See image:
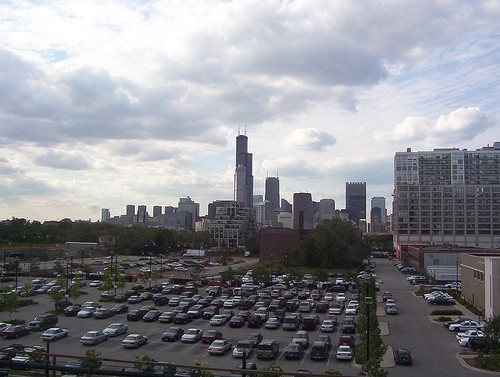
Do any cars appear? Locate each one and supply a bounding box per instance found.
[207,339,232,356]
[10,346,44,364]
[122,333,148,350]
[40,327,69,342]
[357,261,377,279]
[336,335,356,361]
[235,362,313,377]
[102,323,129,337]
[443,317,487,345]
[230,332,263,358]
[383,291,398,316]
[0,322,12,331]
[308,334,333,362]
[423,290,457,306]
[394,348,413,366]
[11,255,361,344]
[78,329,109,346]
[444,281,462,288]
[376,280,384,291]
[0,325,31,340]
[283,330,310,360]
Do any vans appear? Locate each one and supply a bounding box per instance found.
[396,263,416,275]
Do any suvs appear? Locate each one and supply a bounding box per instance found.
[257,338,280,361]
[405,275,425,281]
[28,313,59,332]
